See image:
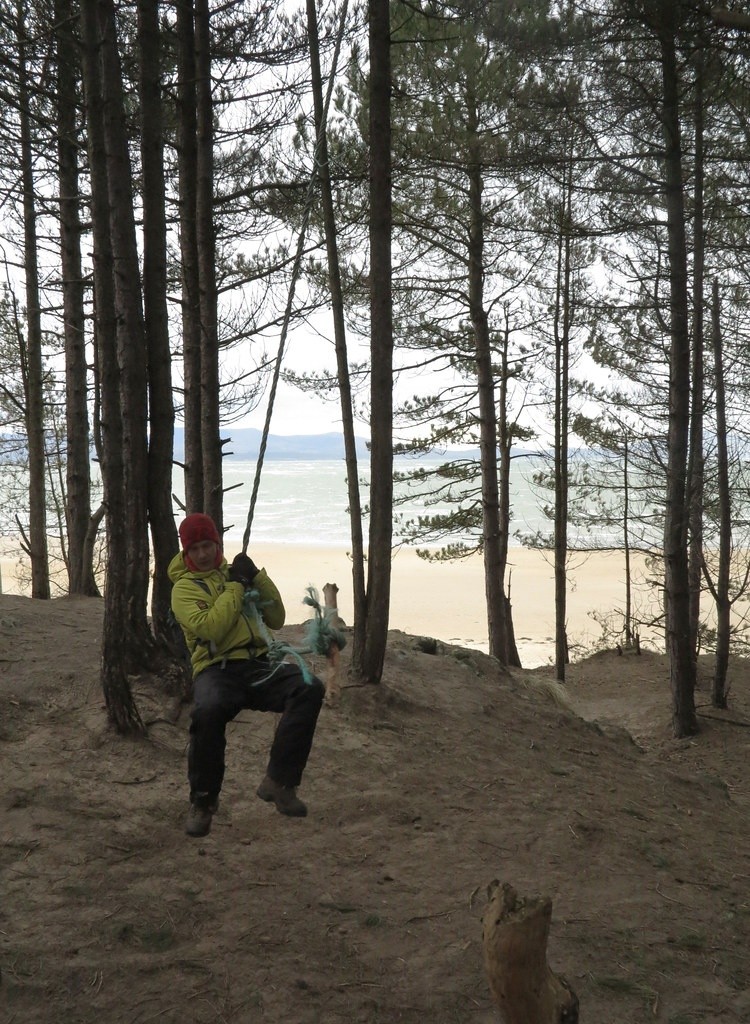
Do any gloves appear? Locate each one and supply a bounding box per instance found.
[233,553,260,579]
[228,565,249,590]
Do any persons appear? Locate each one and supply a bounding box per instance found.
[167,509,330,839]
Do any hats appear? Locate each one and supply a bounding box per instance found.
[178,513,220,552]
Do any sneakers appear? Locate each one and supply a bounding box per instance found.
[185,792,221,839]
[255,775,308,818]
